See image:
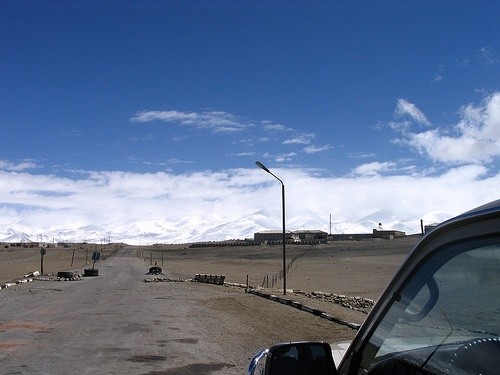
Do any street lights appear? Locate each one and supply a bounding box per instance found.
[255,161,287,295]
